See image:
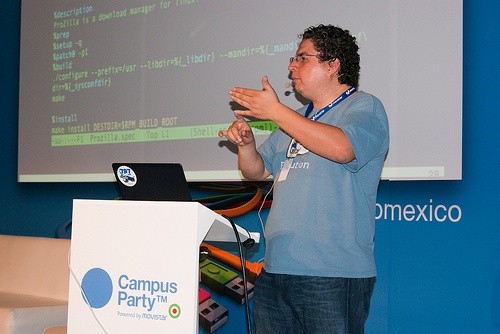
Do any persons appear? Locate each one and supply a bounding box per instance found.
[218,24,390,334]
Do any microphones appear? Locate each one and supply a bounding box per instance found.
[284,82,295,88]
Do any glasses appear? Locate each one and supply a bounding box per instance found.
[290,54,321,64]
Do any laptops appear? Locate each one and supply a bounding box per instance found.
[112,163,192,202]
